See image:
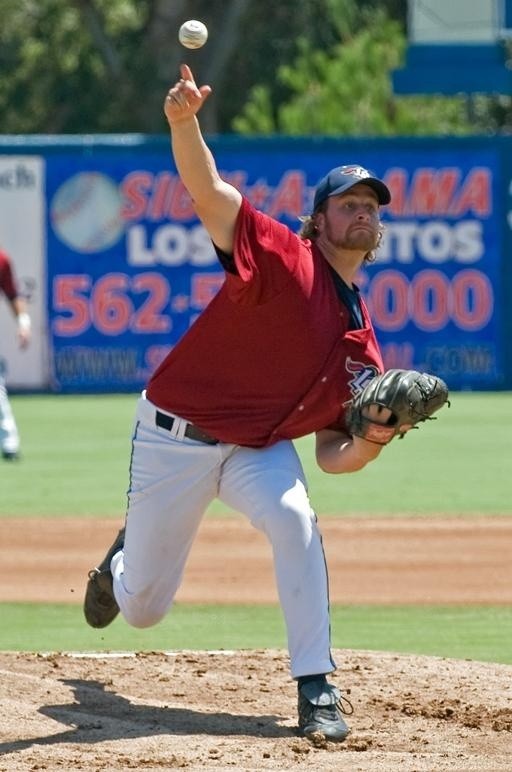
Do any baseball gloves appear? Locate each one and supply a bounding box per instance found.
[346,365,448,445]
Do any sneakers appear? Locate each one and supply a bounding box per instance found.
[84,528,125,629]
[297,681,348,741]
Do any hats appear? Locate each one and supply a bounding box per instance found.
[313,164,392,207]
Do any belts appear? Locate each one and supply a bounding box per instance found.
[155,411,217,445]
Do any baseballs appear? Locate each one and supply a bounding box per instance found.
[178,17,210,50]
[49,171,129,254]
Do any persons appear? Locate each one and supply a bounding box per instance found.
[86,64,450,741]
[2,248,32,459]
